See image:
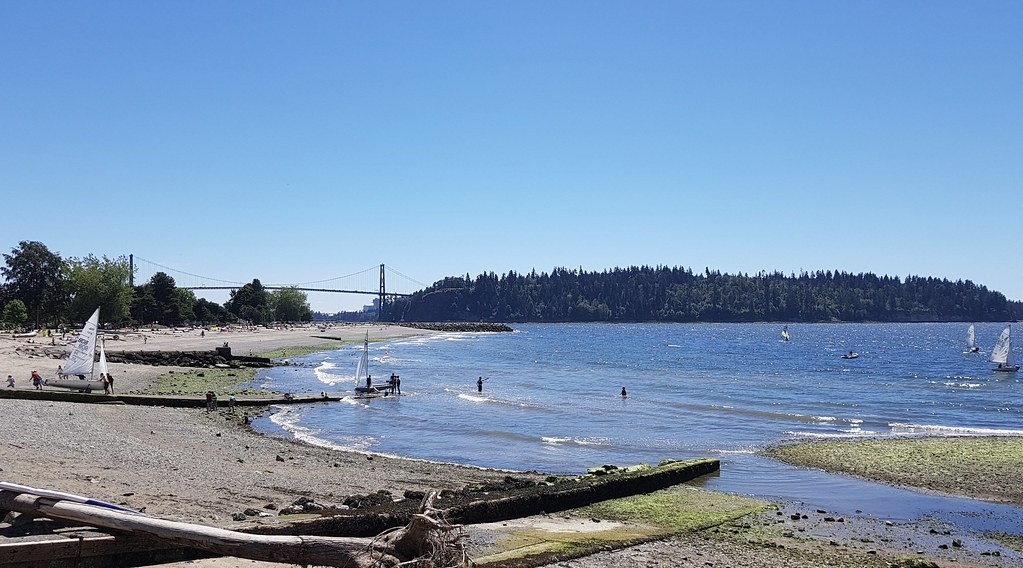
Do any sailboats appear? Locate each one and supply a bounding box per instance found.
[961,323,979,354]
[42,306,112,390]
[353,328,392,391]
[987,323,1022,371]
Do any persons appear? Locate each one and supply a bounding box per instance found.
[284,359,291,366]
[848,350,853,357]
[367,375,372,393]
[389,373,401,395]
[75,374,87,380]
[228,393,236,412]
[267,320,395,331]
[998,363,1008,368]
[55,365,68,380]
[96,373,110,395]
[206,391,218,414]
[786,337,788,341]
[619,387,627,396]
[29,370,45,390]
[6,374,15,388]
[107,373,114,394]
[476,376,483,392]
[5,320,262,345]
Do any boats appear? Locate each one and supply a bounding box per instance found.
[841,352,859,359]
